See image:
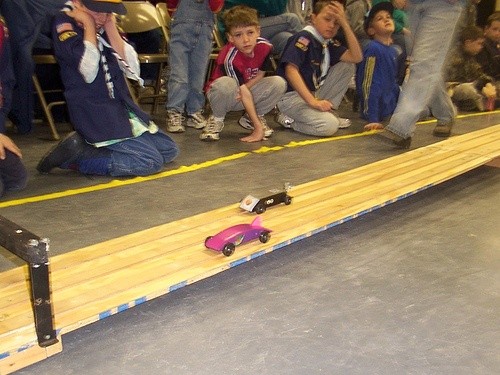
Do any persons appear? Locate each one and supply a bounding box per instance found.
[198,0,500,149]
[1,0,179,199]
[165,0,224,132]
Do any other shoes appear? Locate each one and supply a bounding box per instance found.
[7,107,32,134]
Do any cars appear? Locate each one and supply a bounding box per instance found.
[239,186,293,215]
[204,217,272,257]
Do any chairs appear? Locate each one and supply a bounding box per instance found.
[30,1,222,141]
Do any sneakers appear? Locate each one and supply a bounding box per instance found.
[184,111,207,129]
[273,112,293,129]
[198,114,224,142]
[36,130,93,173]
[239,114,273,137]
[374,129,411,149]
[165,109,186,132]
[433,113,453,137]
[338,116,351,128]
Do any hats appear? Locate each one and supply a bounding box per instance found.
[363,1,393,30]
[81,0,128,15]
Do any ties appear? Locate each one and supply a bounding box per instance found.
[300,24,333,78]
